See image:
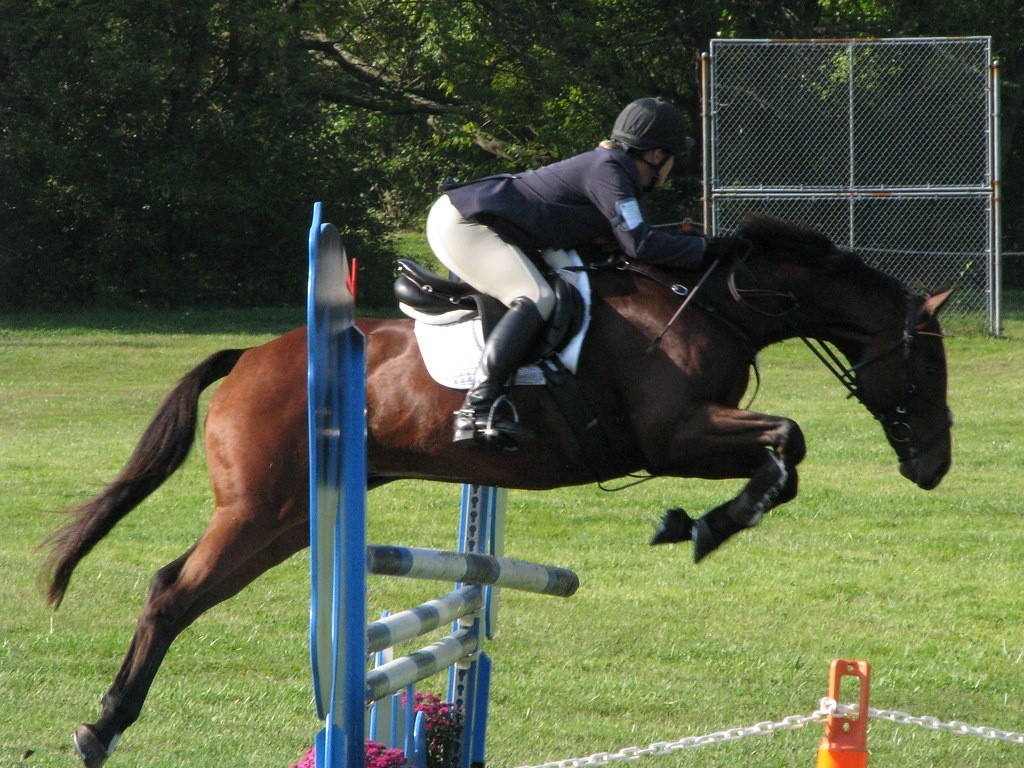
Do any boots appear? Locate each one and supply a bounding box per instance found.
[451,296,536,446]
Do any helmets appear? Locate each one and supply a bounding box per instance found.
[611,95,695,156]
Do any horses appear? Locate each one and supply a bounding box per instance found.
[30,214,955,768]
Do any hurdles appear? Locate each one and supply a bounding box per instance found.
[307,199,578,766]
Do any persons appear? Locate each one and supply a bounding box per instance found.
[427,99,749,445]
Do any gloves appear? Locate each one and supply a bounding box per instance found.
[704,234,750,264]
